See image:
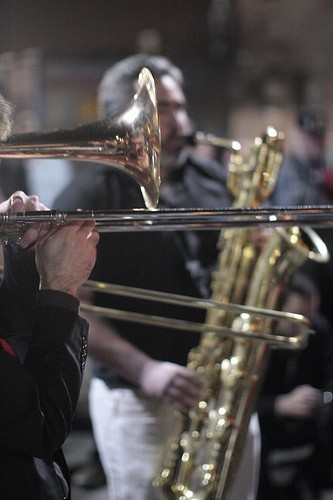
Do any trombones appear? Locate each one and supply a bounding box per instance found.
[73,279,312,350]
[0,67,333,234]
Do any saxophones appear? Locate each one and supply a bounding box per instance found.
[157,125,330,499]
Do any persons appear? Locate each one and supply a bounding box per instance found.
[1,95,102,500]
[197,96,333,500]
[51,51,286,500]
